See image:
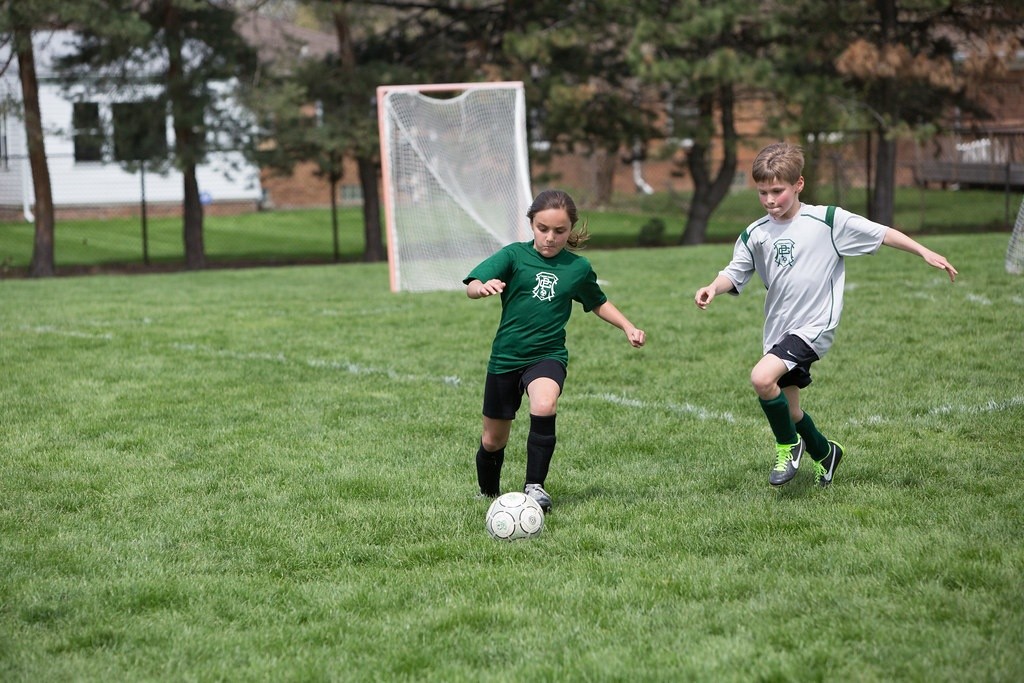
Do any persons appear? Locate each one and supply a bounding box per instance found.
[694,139,959,491]
[463,190,648,508]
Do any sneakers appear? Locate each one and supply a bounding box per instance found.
[813,440,845,487]
[524,483,552,513]
[768,433,806,487]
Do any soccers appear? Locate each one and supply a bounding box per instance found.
[485,492,547,542]
